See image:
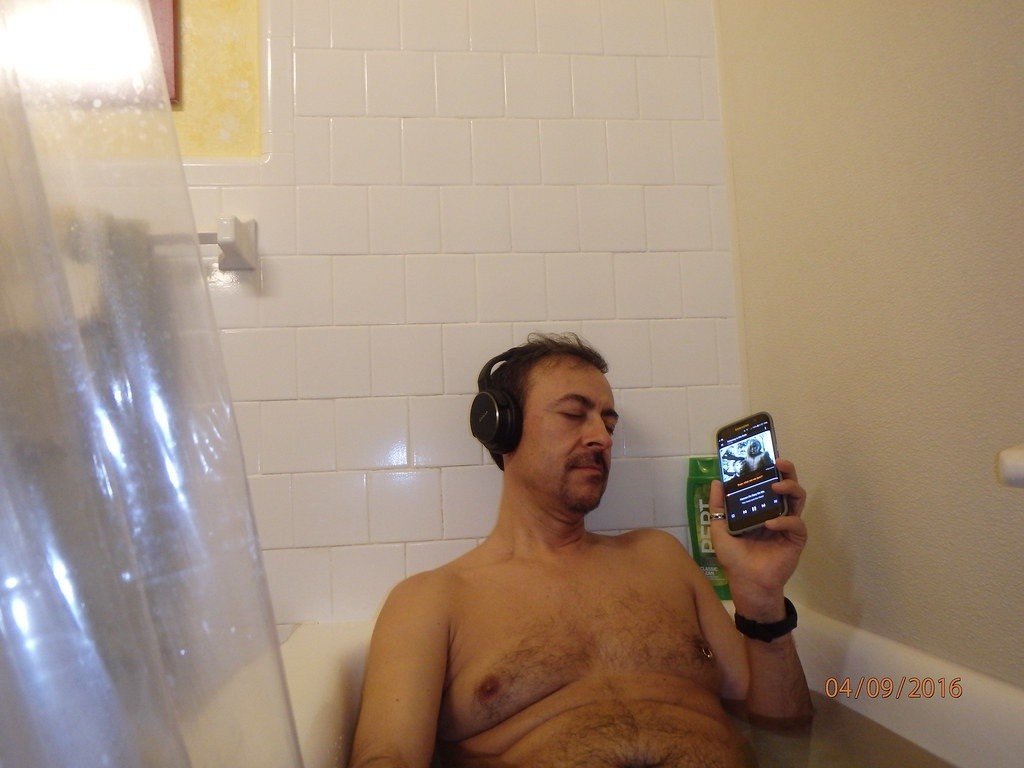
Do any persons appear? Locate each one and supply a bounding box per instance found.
[744,439,771,473]
[351,337,812,768]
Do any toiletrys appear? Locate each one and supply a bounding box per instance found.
[685,455,732,603]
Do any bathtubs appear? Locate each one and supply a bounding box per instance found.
[189,591,1024,768]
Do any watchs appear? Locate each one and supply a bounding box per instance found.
[734,597,797,642]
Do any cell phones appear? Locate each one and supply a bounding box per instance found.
[718,411,790,536]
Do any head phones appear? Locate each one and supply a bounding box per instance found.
[469,346,527,455]
